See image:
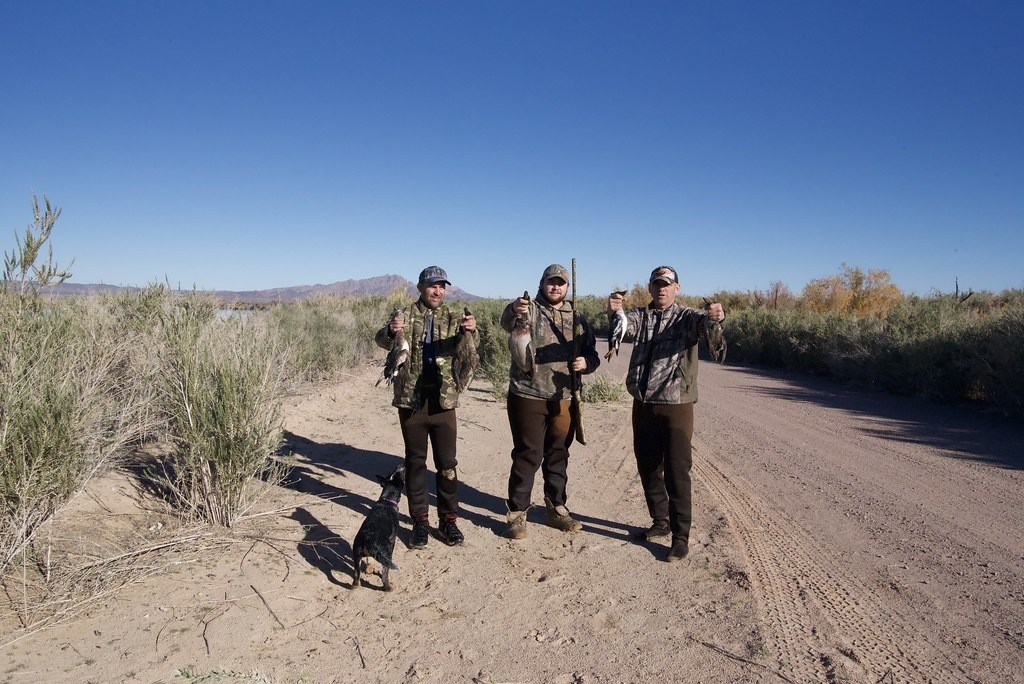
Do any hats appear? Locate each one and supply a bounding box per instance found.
[544,264,568,283]
[651,266,678,284]
[419,265,451,286]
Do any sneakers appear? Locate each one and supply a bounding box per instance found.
[409,512,430,548]
[438,516,465,546]
[629,526,670,544]
[505,500,534,539]
[543,497,582,530]
[667,541,688,562]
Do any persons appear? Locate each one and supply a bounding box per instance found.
[499,263,600,540]
[604,265,726,562]
[373,265,481,549]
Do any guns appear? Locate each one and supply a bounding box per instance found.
[570,258,587,446]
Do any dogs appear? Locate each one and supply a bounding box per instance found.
[350,463,406,593]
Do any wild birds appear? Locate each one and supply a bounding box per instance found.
[507,290,537,381]
[702,297,728,365]
[374,307,410,390]
[604,290,628,363]
[451,307,480,394]
